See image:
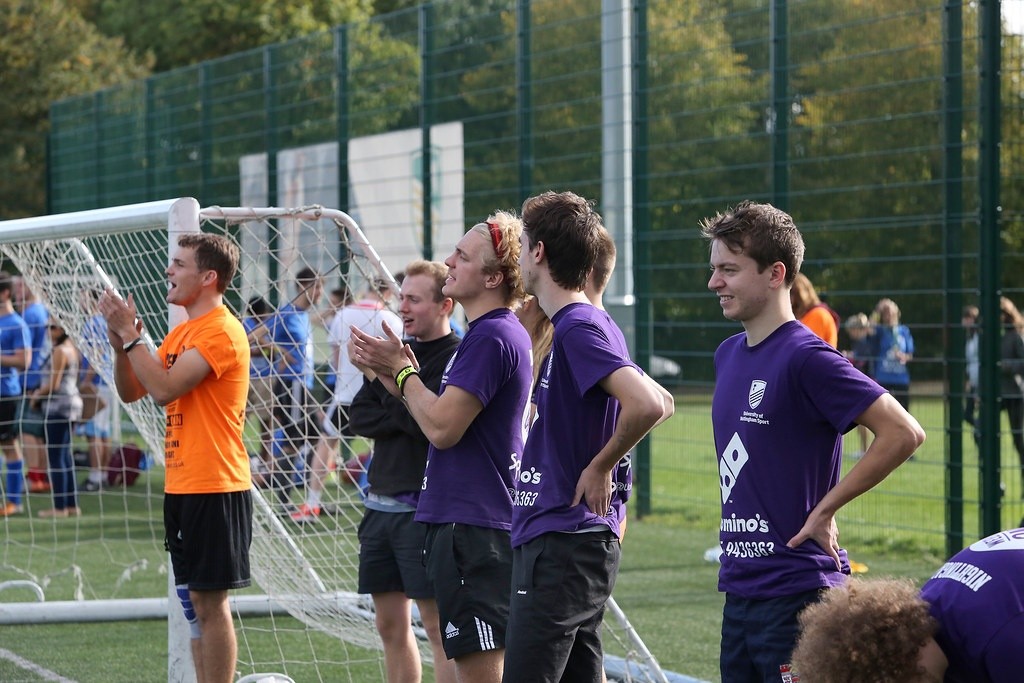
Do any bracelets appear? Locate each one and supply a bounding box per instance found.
[395,365,422,396]
[123,337,145,353]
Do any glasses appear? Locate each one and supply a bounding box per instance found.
[45,323,61,331]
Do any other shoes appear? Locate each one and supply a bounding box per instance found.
[39,503,82,517]
[21,478,50,492]
[78,477,109,491]
[0,502,24,517]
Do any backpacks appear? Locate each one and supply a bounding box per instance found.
[108,442,144,488]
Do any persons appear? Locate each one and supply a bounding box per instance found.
[789,273,915,459]
[501,191,673,683]
[349,209,532,683]
[960,297,1024,527]
[96,233,253,683]
[699,199,926,683]
[0,268,118,518]
[243,267,465,524]
[349,260,463,683]
[790,528,1023,683]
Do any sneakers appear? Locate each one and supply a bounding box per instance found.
[292,504,319,523]
